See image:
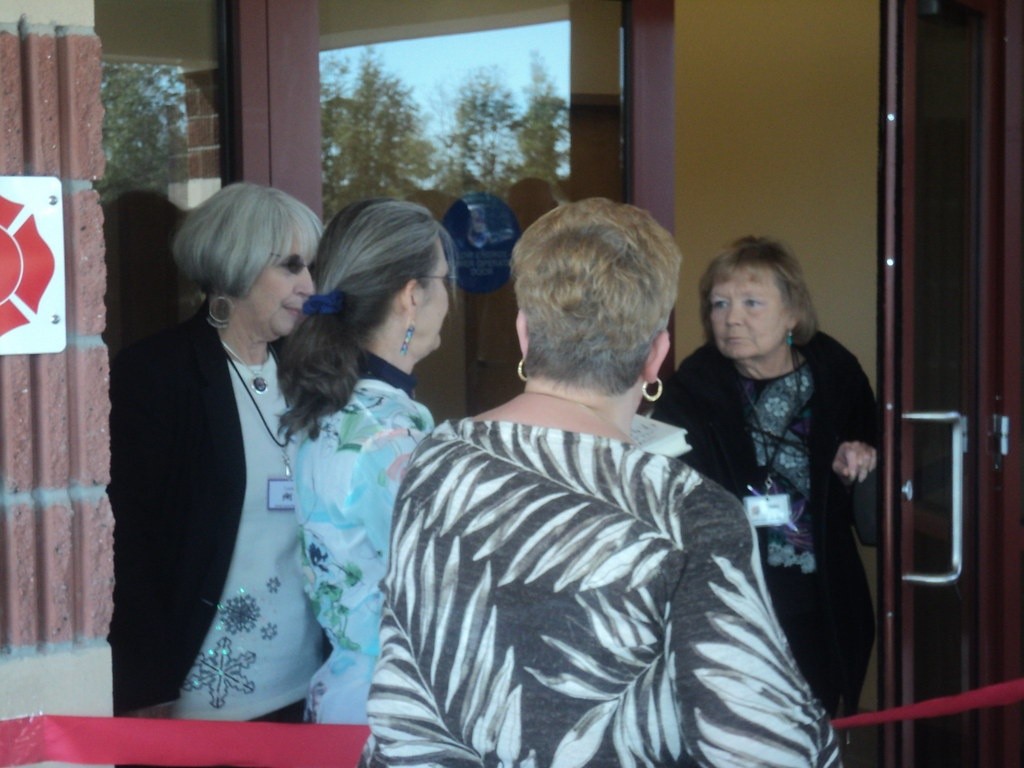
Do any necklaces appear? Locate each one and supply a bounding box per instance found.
[221,337,269,393]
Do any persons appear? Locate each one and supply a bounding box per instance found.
[304,196,848,768]
[109,181,332,768]
[275,197,455,725]
[651,234,883,719]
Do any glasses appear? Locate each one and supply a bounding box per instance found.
[271,250,319,280]
[417,266,460,307]
